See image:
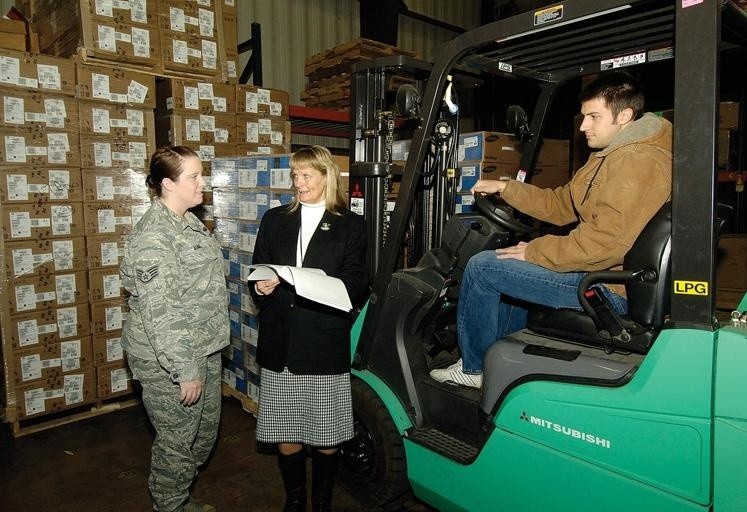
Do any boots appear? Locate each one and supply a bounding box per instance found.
[276,446,307,512]
[310,448,342,512]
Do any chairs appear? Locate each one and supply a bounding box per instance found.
[479,201,734,417]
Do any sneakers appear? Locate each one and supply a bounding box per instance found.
[183,496,217,512]
[427,356,483,388]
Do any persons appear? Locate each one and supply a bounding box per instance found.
[117,143,233,511]
[428,68,673,396]
[246,143,373,510]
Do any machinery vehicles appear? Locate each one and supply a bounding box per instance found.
[335,0,746,512]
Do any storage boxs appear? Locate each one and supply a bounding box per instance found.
[664,99,746,310]
[1,0,569,422]
